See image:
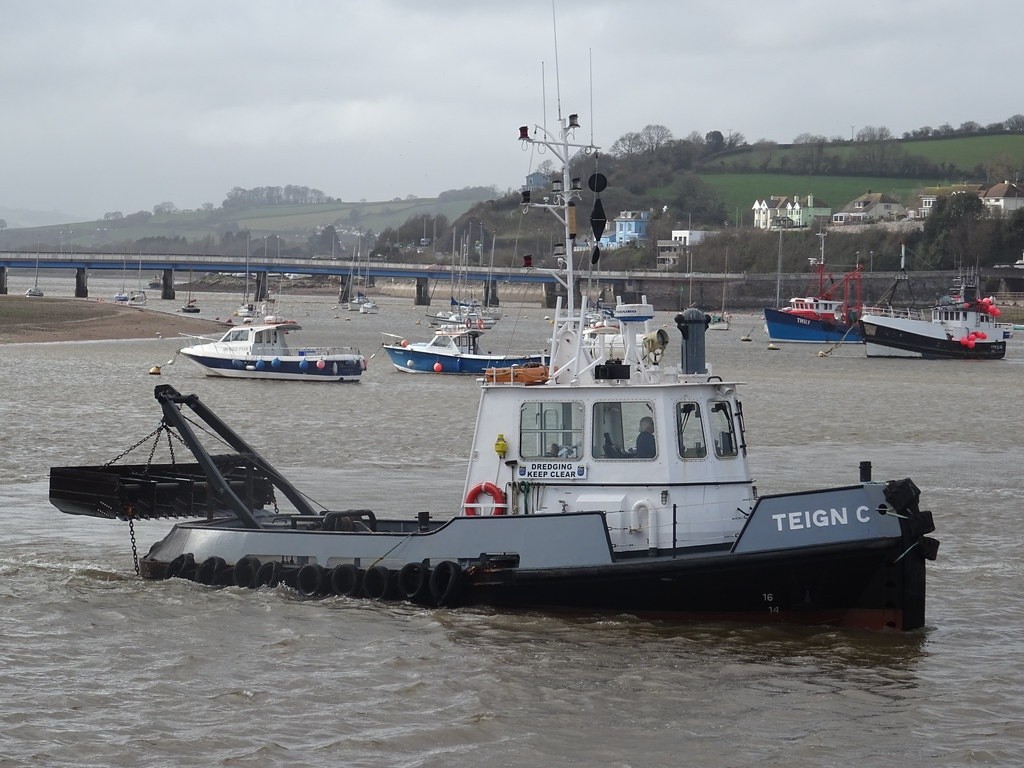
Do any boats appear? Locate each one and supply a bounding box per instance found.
[762,259,867,344]
[48,0,940,630]
[382,300,550,376]
[180,316,367,382]
[857,255,1014,359]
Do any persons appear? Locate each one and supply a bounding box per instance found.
[636,417,656,458]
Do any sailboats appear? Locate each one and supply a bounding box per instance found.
[19,214,751,330]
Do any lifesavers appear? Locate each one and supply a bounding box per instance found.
[658,446,778,547]
[161,552,463,609]
[476,318,483,329]
[465,317,472,329]
[463,481,505,515]
